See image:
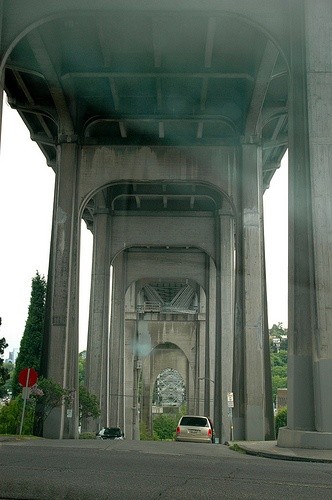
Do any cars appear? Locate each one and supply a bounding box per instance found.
[95,427,124,440]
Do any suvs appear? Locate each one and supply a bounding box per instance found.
[175,415,214,443]
